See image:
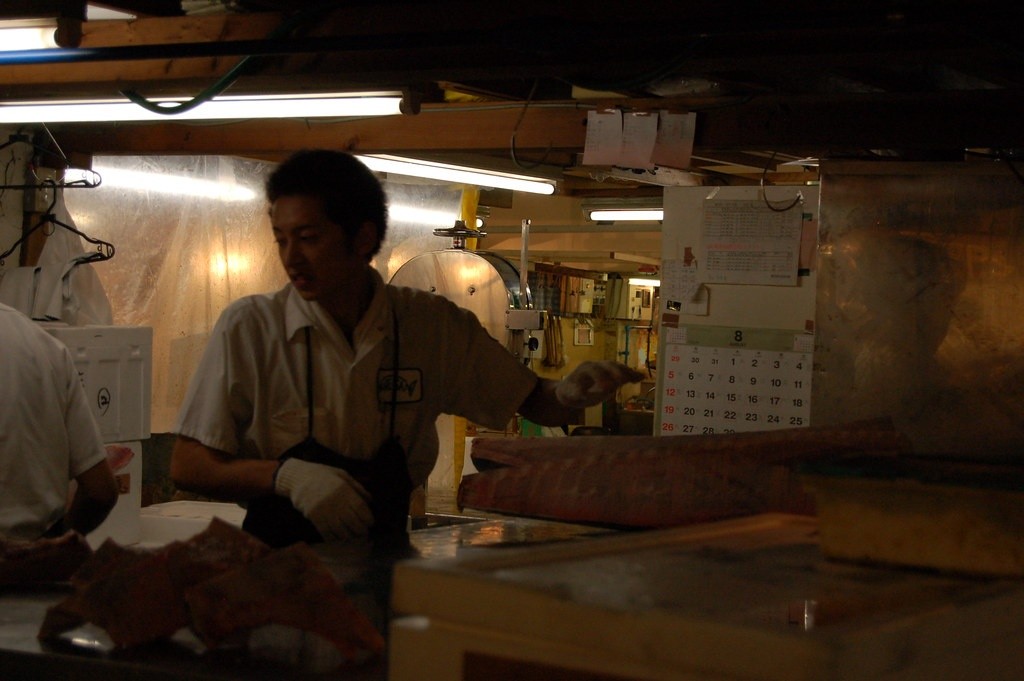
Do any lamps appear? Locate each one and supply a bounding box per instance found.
[354,152,565,198]
[579,203,664,223]
[0,74,422,128]
[0,14,83,51]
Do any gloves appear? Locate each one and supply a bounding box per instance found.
[555,360,645,409]
[275,457,375,543]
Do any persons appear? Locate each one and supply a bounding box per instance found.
[1,300,119,540]
[169,147,647,549]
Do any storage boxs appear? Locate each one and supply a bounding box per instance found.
[42,322,152,444]
[69,442,142,512]
